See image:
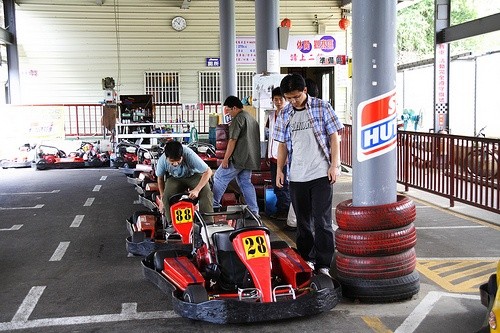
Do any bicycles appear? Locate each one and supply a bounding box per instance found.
[464,126,500,180]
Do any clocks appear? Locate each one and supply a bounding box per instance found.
[172,16,187,31]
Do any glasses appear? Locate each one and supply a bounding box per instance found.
[286,90,303,102]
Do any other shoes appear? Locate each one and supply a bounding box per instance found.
[270,212,280,218]
[281,223,297,232]
[245,216,260,220]
[307,261,314,271]
[277,215,287,220]
[318,268,331,277]
[214,208,220,212]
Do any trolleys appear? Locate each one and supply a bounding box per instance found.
[411,128,450,170]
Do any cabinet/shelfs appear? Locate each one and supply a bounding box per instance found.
[120,95,153,145]
[116,123,195,149]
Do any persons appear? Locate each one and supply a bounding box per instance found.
[155,140,213,225]
[264,87,292,222]
[212,95,261,225]
[273,73,344,279]
